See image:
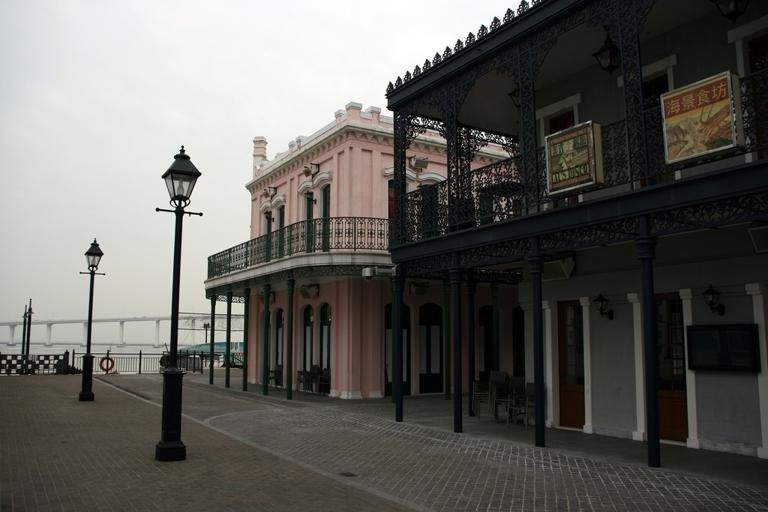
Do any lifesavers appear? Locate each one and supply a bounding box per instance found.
[100,357,114,371]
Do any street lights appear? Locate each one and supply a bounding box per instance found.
[79,238,106,401]
[156,145,204,460]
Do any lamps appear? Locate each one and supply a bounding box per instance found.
[508,87,521,110]
[593,35,621,77]
[593,290,614,320]
[264,209,276,222]
[303,189,316,205]
[701,283,726,316]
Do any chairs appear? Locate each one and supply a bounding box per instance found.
[265,365,281,391]
[470,369,547,432]
[294,370,330,398]
